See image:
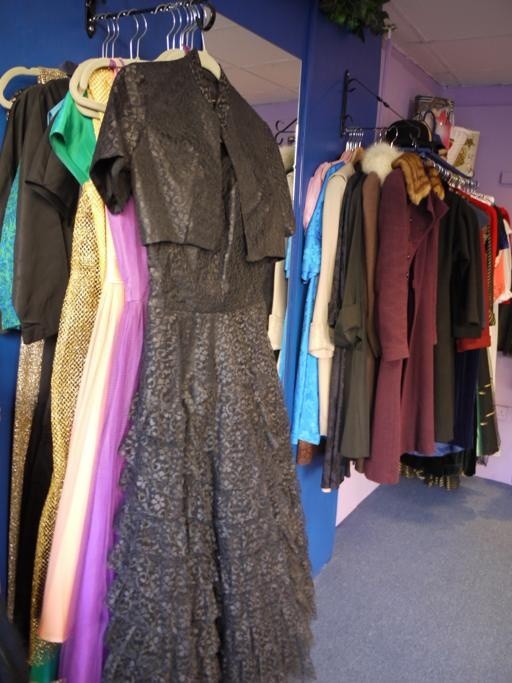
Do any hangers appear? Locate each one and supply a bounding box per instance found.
[345,126,479,194]
[89,0,209,58]
